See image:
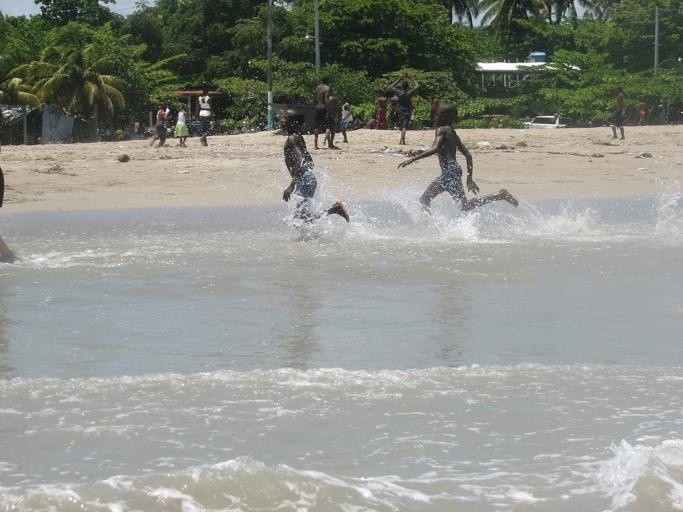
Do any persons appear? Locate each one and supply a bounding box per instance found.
[192,87,215,148]
[608,86,628,142]
[149,105,172,149]
[0,165,14,263]
[395,99,519,220]
[173,104,195,148]
[636,98,648,127]
[276,107,350,245]
[305,72,419,150]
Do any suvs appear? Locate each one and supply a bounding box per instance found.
[523,115,568,128]
